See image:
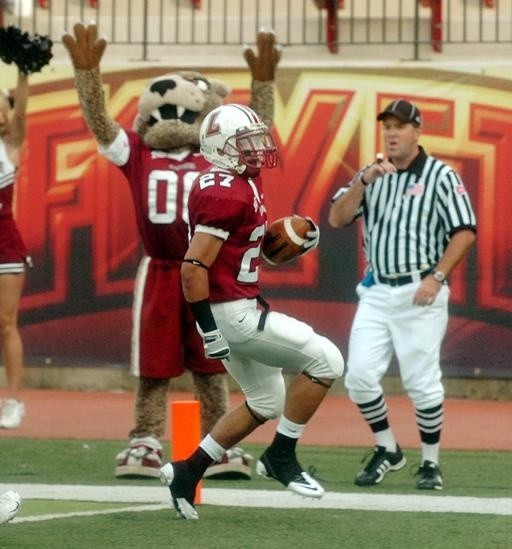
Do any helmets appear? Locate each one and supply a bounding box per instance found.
[198,103,279,177]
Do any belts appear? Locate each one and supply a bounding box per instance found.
[375,270,435,289]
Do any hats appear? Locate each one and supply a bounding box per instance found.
[376,99,422,126]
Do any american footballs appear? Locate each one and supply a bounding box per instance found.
[260,217,311,267]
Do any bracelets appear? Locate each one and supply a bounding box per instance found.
[361,176,367,186]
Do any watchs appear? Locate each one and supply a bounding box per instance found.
[428,268,447,286]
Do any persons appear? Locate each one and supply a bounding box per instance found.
[1,488,24,526]
[155,99,347,522]
[1,67,36,431]
[324,99,482,496]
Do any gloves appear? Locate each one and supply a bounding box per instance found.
[293,212,321,258]
[200,328,232,362]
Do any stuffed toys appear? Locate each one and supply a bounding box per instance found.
[60,19,284,481]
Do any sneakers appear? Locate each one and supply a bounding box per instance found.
[159,461,201,520]
[0,489,21,524]
[255,446,325,499]
[354,443,407,487]
[203,447,252,481]
[414,459,443,490]
[0,397,25,429]
[113,436,164,478]
